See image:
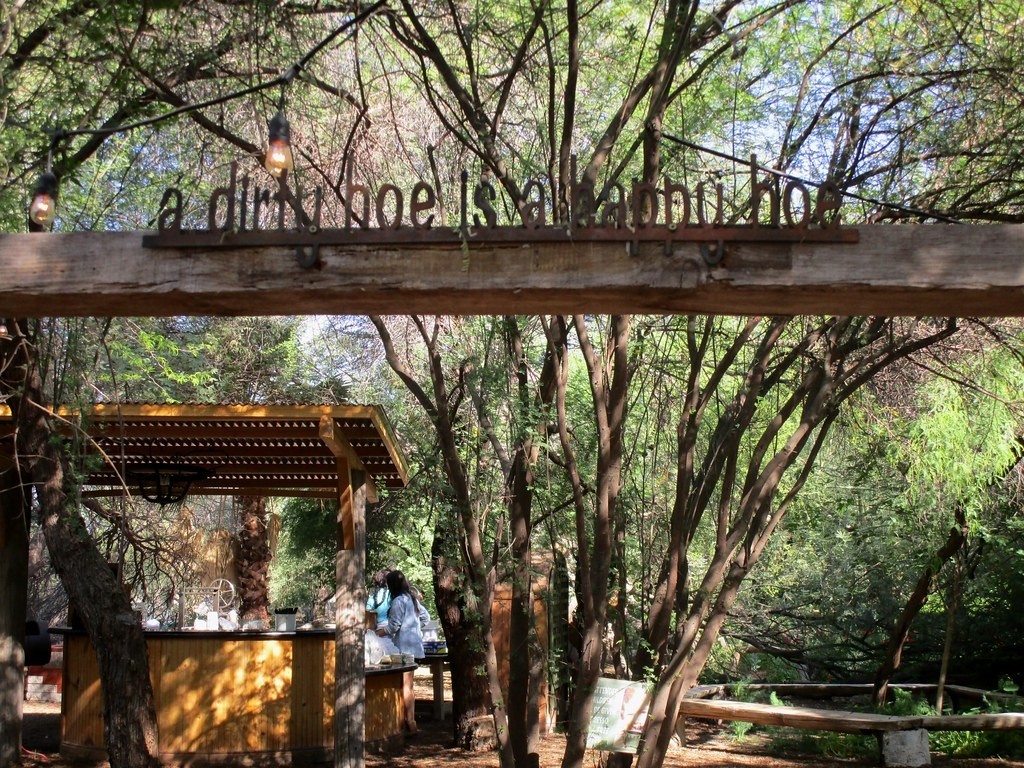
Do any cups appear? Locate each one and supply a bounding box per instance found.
[207,611,218,630]
[275,614,296,631]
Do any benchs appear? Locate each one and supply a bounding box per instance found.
[675,683,1024,768]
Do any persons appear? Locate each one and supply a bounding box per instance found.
[365,570,430,736]
[227,607,239,631]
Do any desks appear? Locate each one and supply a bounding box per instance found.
[365,662,418,675]
[424,654,453,720]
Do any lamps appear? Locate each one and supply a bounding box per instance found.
[125,463,211,507]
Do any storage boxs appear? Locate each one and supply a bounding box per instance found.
[423,641,448,654]
